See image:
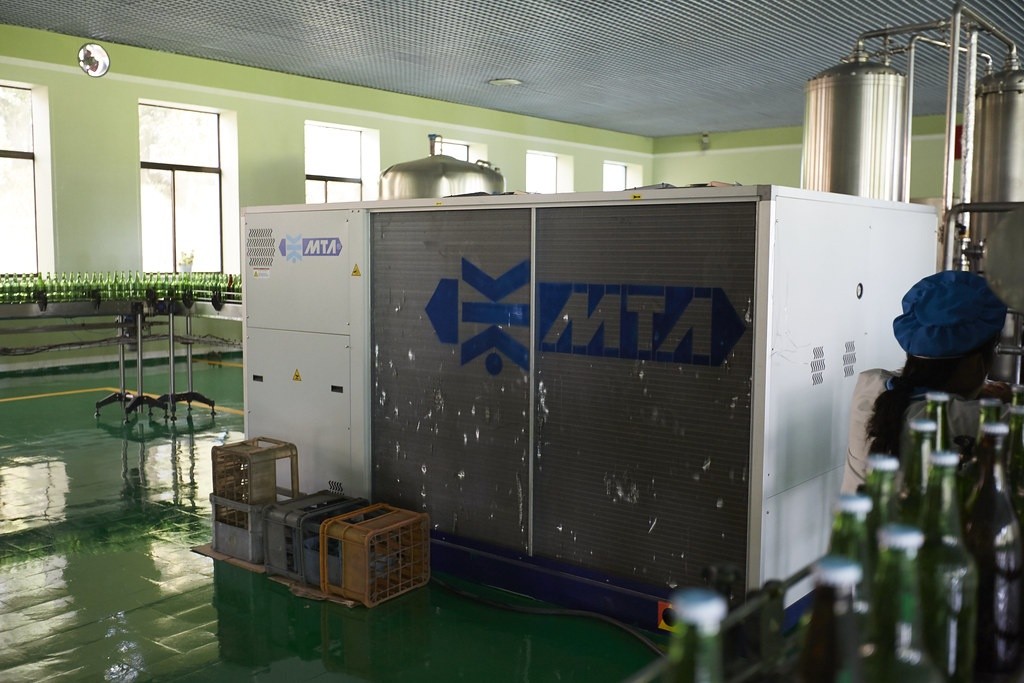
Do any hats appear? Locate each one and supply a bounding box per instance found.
[892,271,1007,358]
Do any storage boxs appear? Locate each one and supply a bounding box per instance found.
[211,437,431,609]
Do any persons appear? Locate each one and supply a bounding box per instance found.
[838,268,1013,496]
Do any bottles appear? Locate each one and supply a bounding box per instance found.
[865,419,935,524]
[193,272,242,300]
[974,398,1002,457]
[36,272,45,302]
[28,273,36,303]
[53,272,61,302]
[98,272,105,301]
[119,271,141,299]
[142,272,192,299]
[45,272,53,302]
[803,560,863,683]
[76,272,83,302]
[61,272,68,302]
[669,589,727,683]
[20,273,28,302]
[68,272,76,302]
[0,273,20,303]
[965,423,1024,683]
[925,393,951,454]
[920,451,977,683]
[90,272,98,301]
[105,271,120,300]
[1006,385,1024,543]
[83,272,90,301]
[826,496,943,683]
